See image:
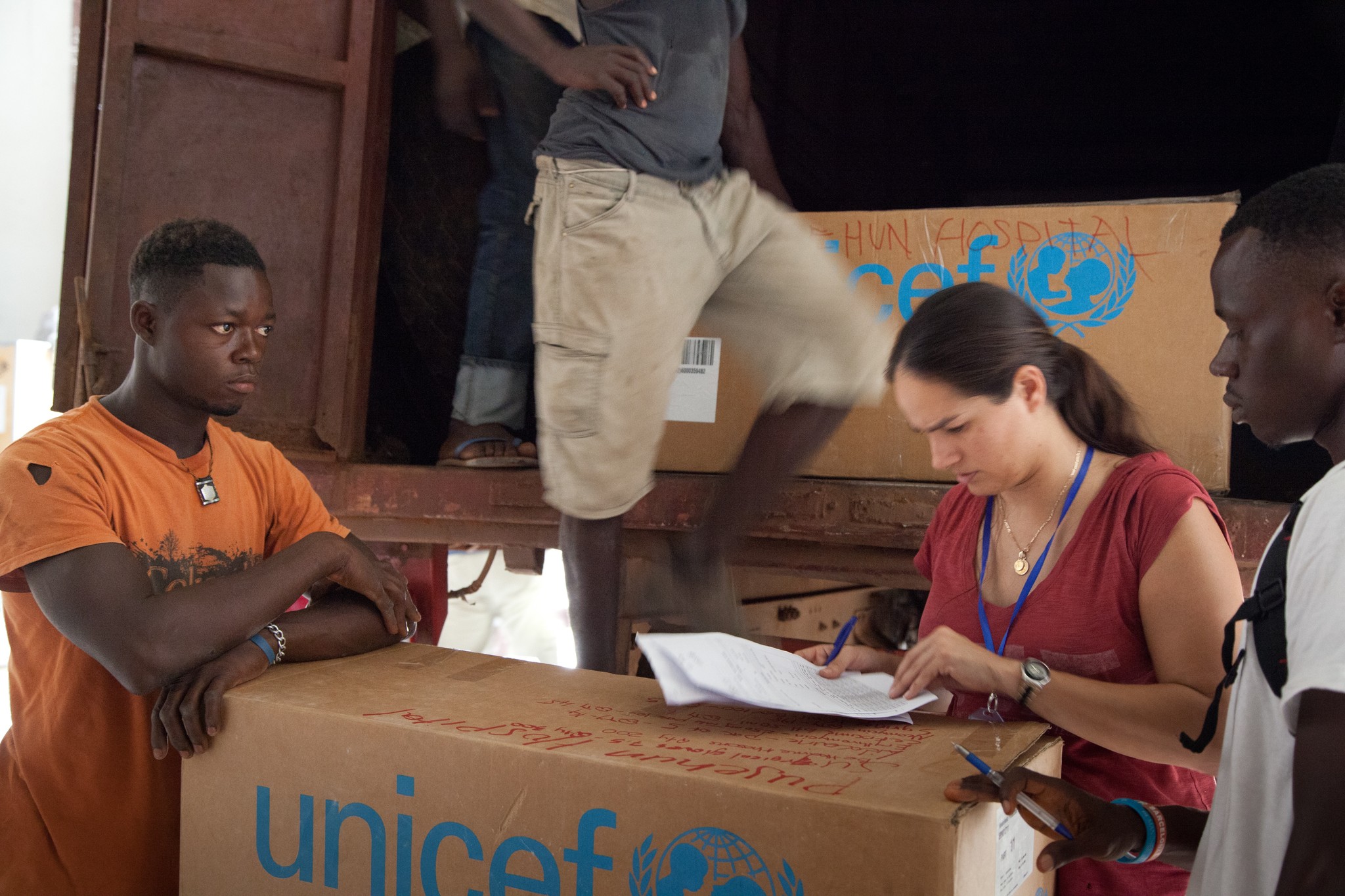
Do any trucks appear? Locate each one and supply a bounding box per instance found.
[67,1,1292,687]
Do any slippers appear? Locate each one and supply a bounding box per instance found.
[432,435,539,471]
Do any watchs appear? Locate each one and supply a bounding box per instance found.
[1016,656,1051,707]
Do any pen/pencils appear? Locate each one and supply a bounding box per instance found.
[950,740,1073,840]
[822,609,861,667]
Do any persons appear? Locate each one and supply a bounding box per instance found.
[793,282,1246,896]
[941,161,1345,896]
[0,217,424,896]
[424,0,580,467]
[458,0,888,675]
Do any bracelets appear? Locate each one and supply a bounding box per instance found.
[1127,800,1166,863]
[1109,798,1156,864]
[251,634,276,667]
[266,620,287,666]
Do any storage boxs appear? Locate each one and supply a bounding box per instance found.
[184,636,1064,896]
[648,196,1238,498]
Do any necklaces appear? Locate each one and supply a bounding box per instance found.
[169,428,220,506]
[997,437,1080,575]
